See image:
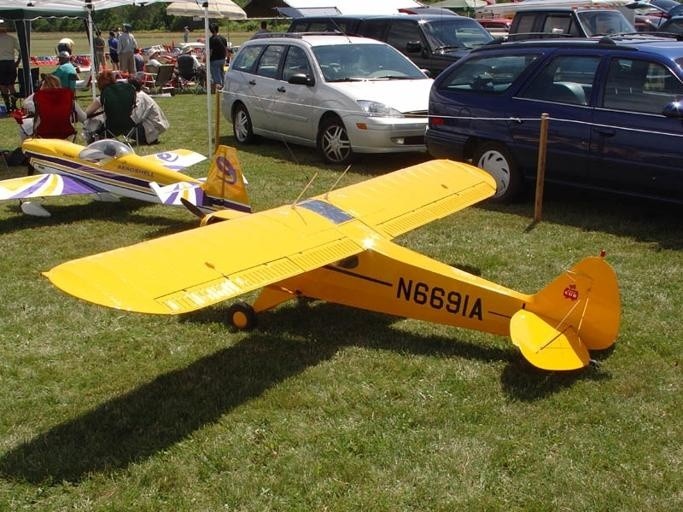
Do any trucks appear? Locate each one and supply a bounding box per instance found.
[481,0,627,18]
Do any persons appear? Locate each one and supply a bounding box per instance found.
[0,23,272,141]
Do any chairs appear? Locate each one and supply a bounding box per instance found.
[335,48,369,79]
[178,56,207,95]
[605,65,657,115]
[32,86,78,144]
[141,64,175,95]
[11,66,39,116]
[100,83,140,146]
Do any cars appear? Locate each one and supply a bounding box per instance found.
[423,30,683,204]
[221,29,439,164]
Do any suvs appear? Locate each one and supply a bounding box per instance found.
[507,7,638,41]
[474,17,512,40]
[627,0,682,38]
[287,15,495,78]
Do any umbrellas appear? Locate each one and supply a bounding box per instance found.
[165,0,247,20]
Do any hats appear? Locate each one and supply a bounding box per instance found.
[55,50,69,59]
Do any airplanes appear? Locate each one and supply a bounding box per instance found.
[45,158,621,389]
[0,136,253,227]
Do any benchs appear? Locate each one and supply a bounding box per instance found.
[523,63,588,106]
